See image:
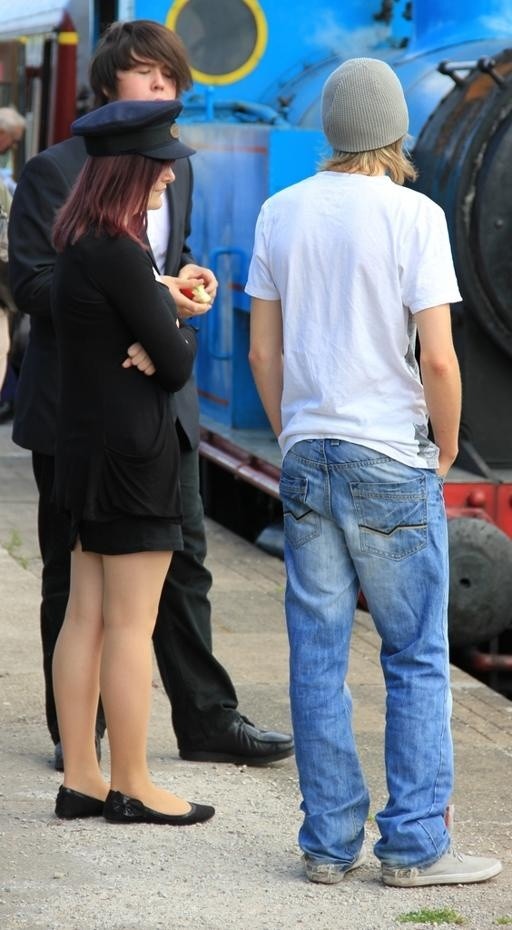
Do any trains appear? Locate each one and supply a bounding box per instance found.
[2,2,512,671]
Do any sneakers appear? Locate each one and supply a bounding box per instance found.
[382,847,504,887]
[304,846,367,884]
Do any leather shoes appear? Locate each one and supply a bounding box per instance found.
[55,734,101,771]
[179,717,295,766]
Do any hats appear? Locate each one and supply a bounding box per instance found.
[321,58,409,153]
[71,100,197,161]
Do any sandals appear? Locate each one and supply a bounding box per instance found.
[55,784,215,825]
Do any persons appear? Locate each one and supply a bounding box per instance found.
[241,55,503,888]
[8,18,298,767]
[0,102,28,428]
[48,95,218,827]
